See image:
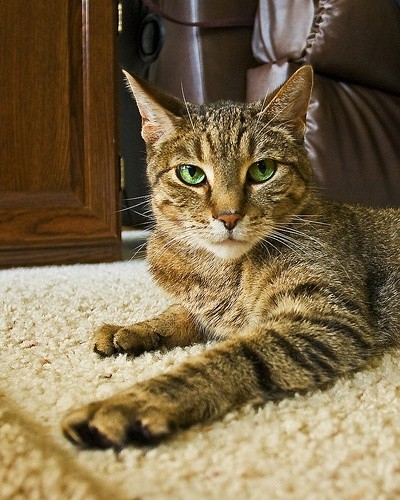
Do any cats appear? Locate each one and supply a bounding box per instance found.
[60,63,400,453]
